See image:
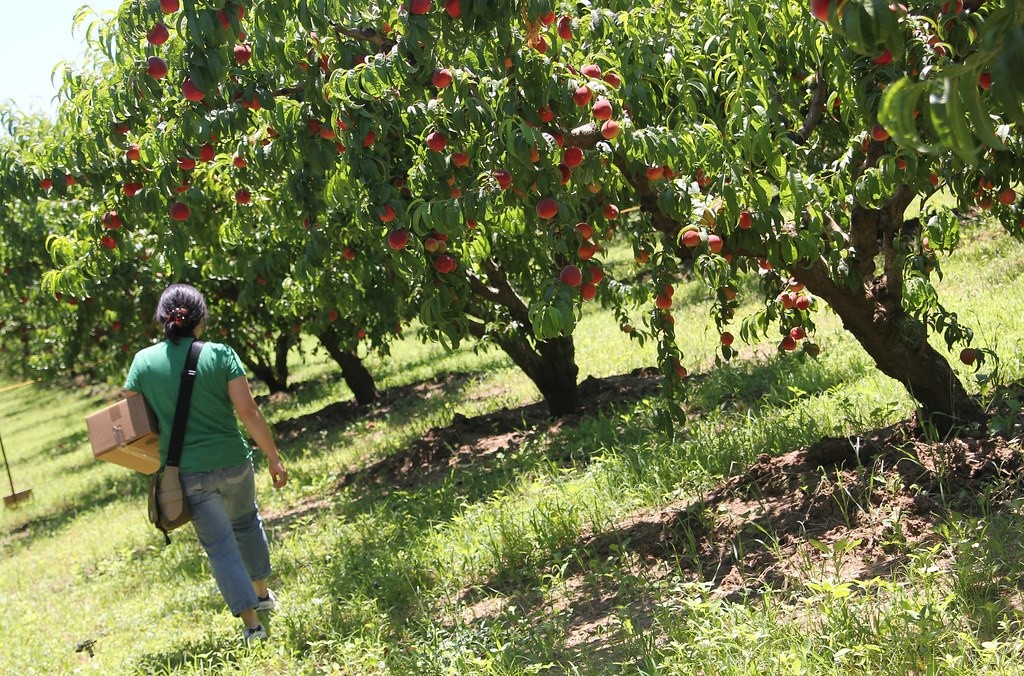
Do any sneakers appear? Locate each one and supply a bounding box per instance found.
[244,588,275,644]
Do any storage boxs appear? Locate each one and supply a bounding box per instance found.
[85,395,163,475]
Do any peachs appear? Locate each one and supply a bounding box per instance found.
[9,1,1024,379]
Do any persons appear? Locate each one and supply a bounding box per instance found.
[121,283,292,648]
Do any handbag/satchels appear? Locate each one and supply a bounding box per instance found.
[147,465,192,545]
